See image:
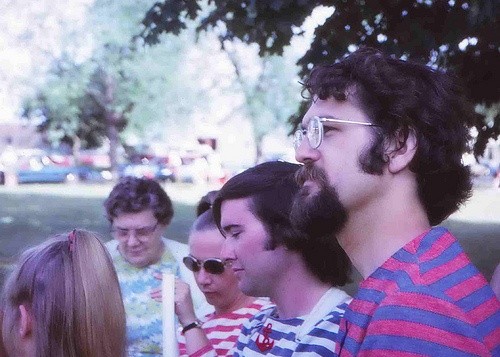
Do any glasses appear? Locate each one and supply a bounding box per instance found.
[108,221,160,240]
[292,116,377,152]
[182,256,227,275]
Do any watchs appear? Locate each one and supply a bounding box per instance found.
[180,319,205,335]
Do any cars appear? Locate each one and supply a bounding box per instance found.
[8,146,198,184]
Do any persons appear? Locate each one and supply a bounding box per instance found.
[0,228,128,357]
[212,160,355,357]
[149,190,278,357]
[100,176,215,357]
[289,46,500,357]
